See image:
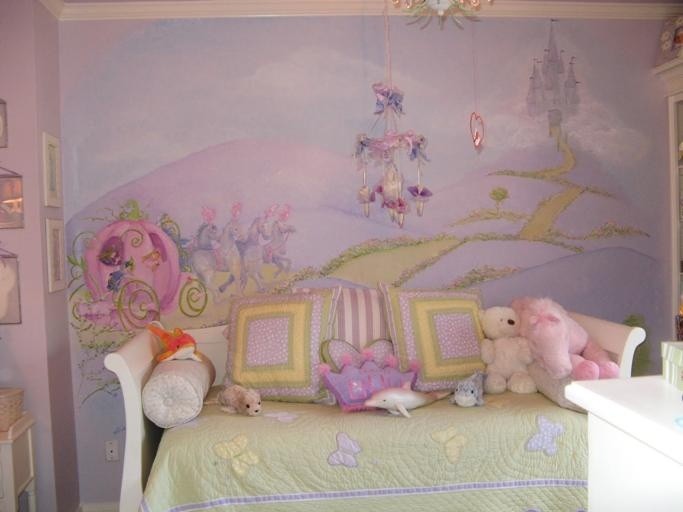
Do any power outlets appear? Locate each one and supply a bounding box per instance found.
[105,441,119,462]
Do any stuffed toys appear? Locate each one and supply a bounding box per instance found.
[363,382,451,418]
[511,295,618,381]
[476,306,538,395]
[144,322,203,364]
[448,369,490,409]
[215,383,263,417]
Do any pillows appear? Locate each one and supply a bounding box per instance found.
[223,285,342,406]
[292,278,392,352]
[141,351,216,428]
[378,282,483,392]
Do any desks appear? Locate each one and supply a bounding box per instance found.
[564,374,683,512]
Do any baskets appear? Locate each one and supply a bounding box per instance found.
[0,386,26,435]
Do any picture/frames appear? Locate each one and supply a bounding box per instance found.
[0,256,21,325]
[656,16,683,66]
[41,132,62,209]
[45,218,65,293]
[0,174,24,228]
[0,102,8,148]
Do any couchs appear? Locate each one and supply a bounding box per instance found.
[103,313,646,512]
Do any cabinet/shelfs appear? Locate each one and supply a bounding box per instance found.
[654,57,683,341]
[0,411,38,511]
